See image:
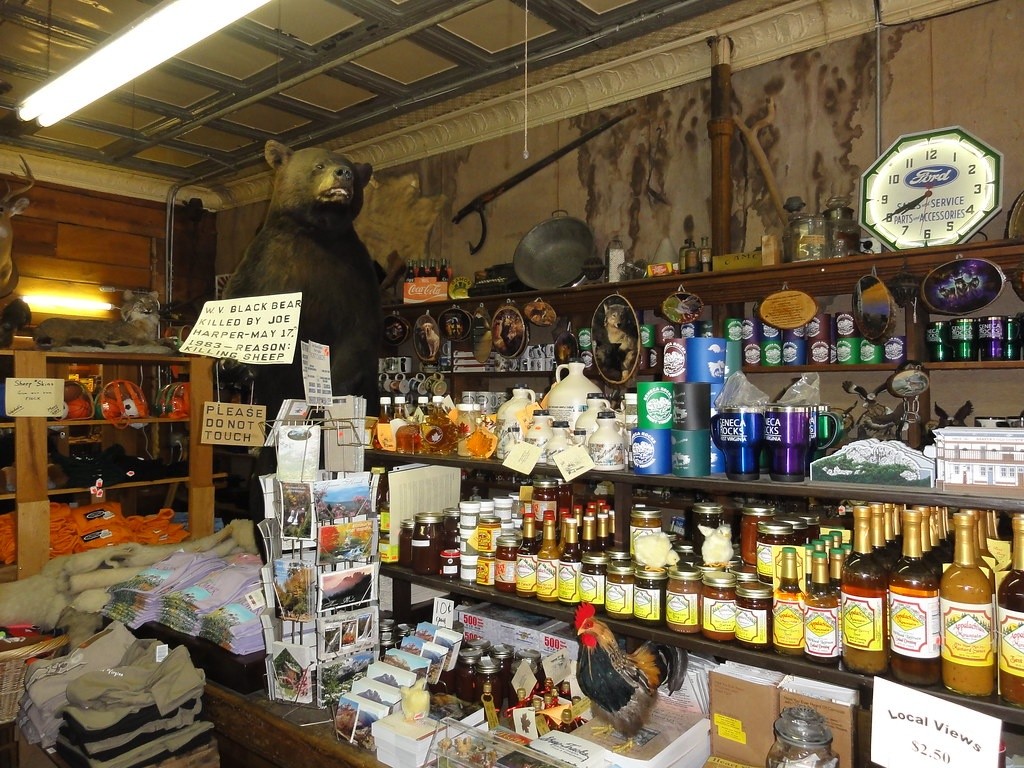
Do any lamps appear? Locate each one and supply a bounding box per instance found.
[0,0,271,137]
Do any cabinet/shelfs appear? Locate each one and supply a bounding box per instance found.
[0,349,229,585]
[378,237,1024,729]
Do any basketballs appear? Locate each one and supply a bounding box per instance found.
[66,400,92,420]
[95,398,121,420]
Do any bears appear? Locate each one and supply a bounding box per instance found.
[218,142,401,534]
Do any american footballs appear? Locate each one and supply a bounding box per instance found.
[105,384,129,400]
[64,380,83,400]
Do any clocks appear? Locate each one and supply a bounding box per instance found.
[858,125,1004,252]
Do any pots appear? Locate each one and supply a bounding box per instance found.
[513,208,594,291]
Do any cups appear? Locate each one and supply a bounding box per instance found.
[576,311,907,479]
[926,311,1024,362]
[462,342,556,410]
[374,354,447,395]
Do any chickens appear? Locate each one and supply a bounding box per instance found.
[572,604,687,755]
[632,531,680,572]
[701,525,734,568]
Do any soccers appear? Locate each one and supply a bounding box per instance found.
[52,401,69,421]
[123,399,140,418]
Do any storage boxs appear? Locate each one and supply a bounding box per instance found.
[702,662,880,768]
[403,267,451,303]
[458,602,580,661]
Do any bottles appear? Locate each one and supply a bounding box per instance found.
[405,257,452,283]
[678,236,713,275]
[365,460,1024,767]
[783,199,861,263]
[378,362,629,471]
